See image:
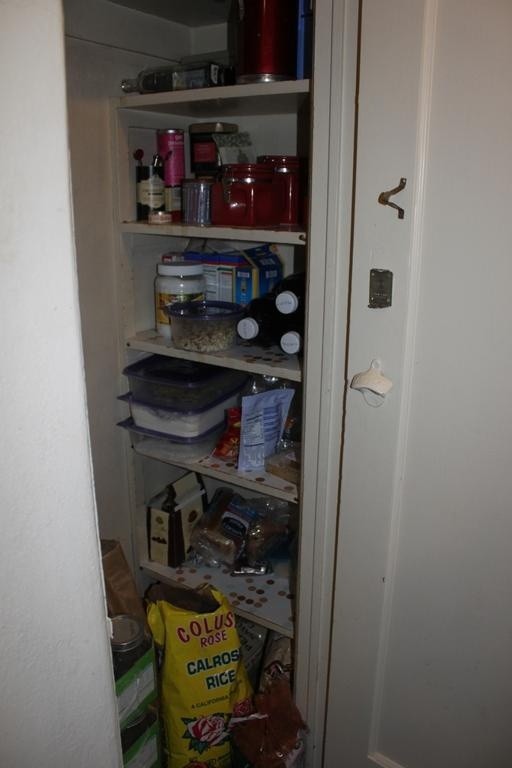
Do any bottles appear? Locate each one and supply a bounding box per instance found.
[111,614,147,679]
[237,299,281,348]
[190,122,238,170]
[273,275,304,318]
[156,261,205,340]
[122,59,222,92]
[262,154,307,231]
[209,164,273,228]
[123,712,148,751]
[183,179,212,227]
[279,319,302,354]
[159,130,185,187]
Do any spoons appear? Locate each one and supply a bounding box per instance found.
[132,148,146,167]
